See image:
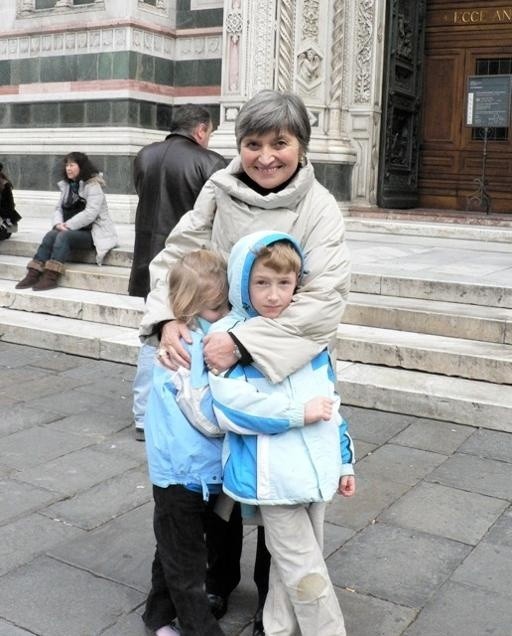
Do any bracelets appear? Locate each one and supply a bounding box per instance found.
[233,344,242,360]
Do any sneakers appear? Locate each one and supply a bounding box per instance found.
[152,623,180,636]
[252,608,263,635]
[205,592,230,618]
[135,429,145,441]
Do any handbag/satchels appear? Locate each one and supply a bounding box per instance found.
[62,201,92,230]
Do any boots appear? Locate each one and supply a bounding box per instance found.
[15,260,66,291]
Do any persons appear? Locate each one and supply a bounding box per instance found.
[138,90,352,636]
[15,152,107,291]
[209,230,355,635]
[0,161,22,242]
[141,250,229,636]
[127,103,228,442]
